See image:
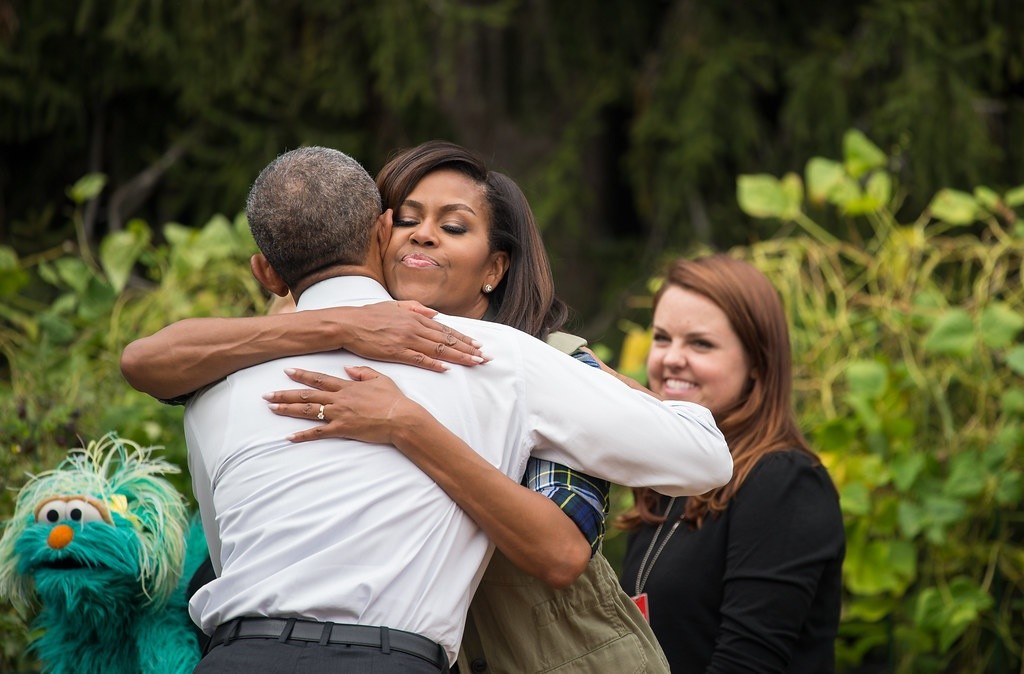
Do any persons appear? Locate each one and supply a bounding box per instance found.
[183,144,733,674]
[120,132,672,674]
[618,255,845,674]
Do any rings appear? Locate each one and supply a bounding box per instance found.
[318,405,325,420]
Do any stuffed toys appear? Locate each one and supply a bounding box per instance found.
[0,432,210,674]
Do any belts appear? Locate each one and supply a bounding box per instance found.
[207,618,450,674]
